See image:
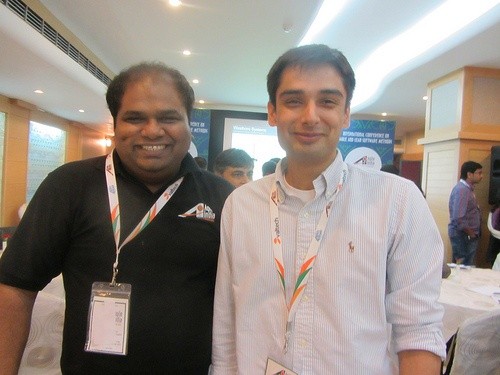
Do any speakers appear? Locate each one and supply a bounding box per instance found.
[488,145,500,205]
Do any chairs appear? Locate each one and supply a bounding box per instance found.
[440,312,500,375]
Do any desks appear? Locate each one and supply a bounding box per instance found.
[440,264,500,336]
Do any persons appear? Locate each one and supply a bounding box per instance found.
[448,161,484,266]
[0,61,236,375]
[214,148,254,188]
[262,158,281,177]
[208,44,447,375]
